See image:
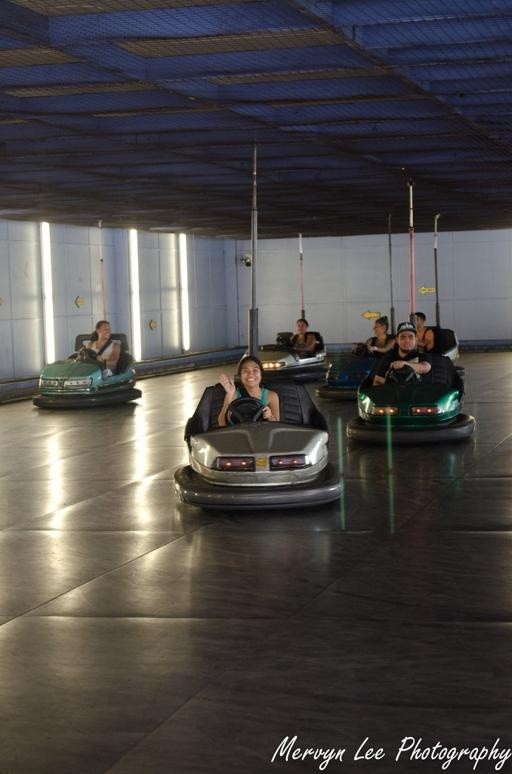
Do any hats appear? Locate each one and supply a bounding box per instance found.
[396,322,416,337]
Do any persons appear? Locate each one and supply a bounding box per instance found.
[217,355,280,427]
[78,320,121,376]
[365,312,434,387]
[290,319,316,359]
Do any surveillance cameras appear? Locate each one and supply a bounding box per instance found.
[242,256,250,267]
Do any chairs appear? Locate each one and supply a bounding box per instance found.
[373,352,456,395]
[74,335,127,373]
[203,382,313,429]
